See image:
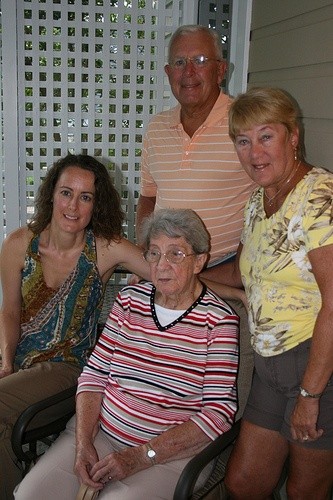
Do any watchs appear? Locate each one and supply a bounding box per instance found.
[146,442,155,466]
[297,387,320,400]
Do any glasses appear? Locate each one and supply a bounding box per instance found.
[140,250,196,264]
[168,55,220,69]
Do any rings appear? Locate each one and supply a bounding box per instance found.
[303,435,310,442]
[108,473,112,481]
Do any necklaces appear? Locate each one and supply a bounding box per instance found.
[264,156,302,207]
[175,124,207,157]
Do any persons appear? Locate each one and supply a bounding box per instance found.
[13,207,242,500]
[128,24,264,422]
[198,87,333,500]
[0,153,248,500]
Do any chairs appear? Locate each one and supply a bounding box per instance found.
[10,266,257,500]
